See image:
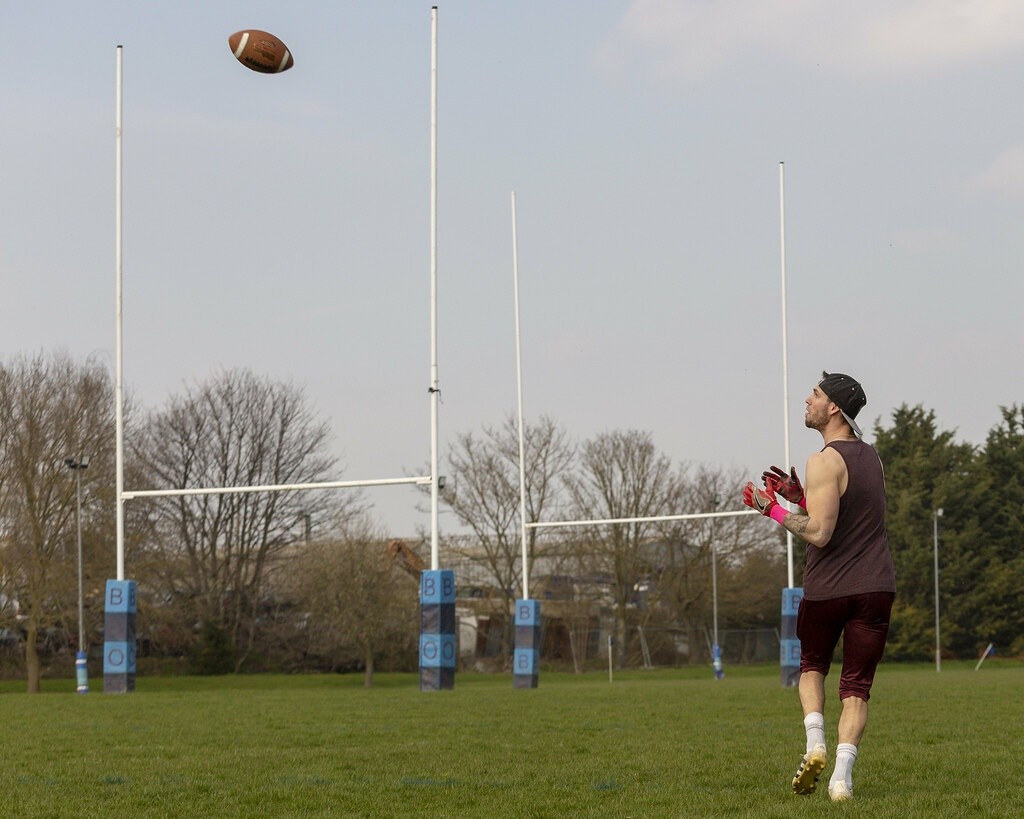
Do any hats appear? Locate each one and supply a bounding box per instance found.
[817,370,869,436]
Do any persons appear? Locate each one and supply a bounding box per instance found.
[741,370,895,802]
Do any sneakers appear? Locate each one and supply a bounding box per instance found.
[825,779,854,802]
[791,744,828,794]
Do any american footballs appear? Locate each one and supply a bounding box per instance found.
[229,28,296,76]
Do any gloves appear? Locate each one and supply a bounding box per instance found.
[760,465,806,512]
[742,475,791,526]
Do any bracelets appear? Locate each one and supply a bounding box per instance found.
[798,496,806,510]
[769,504,792,525]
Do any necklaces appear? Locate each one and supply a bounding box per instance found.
[825,435,857,444]
